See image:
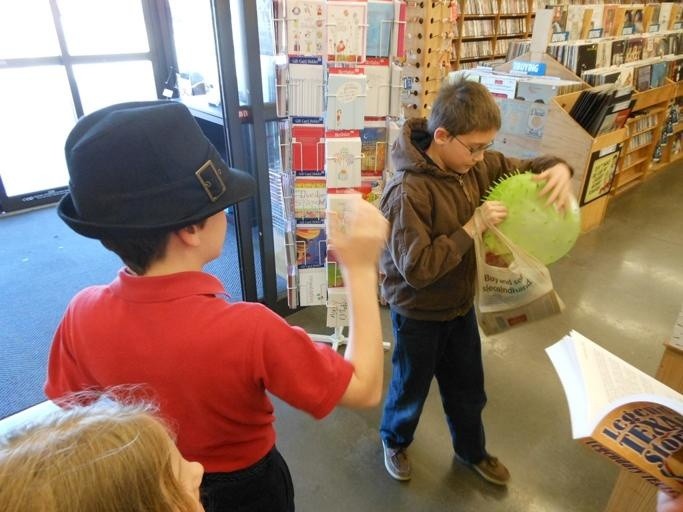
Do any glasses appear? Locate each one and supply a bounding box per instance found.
[455,135,493,154]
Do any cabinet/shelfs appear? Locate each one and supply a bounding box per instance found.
[261,0,683,355]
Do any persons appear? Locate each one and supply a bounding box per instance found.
[656,488,681,511]
[42,97,390,512]
[378,70,574,487]
[0,381,206,511]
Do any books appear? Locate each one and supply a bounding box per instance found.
[544,329,682,512]
[447,1,682,160]
[476,288,561,337]
[266,0,406,329]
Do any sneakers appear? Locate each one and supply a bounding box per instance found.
[454,452,510,486]
[382,439,411,481]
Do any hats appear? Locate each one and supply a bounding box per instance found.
[57,101,258,236]
